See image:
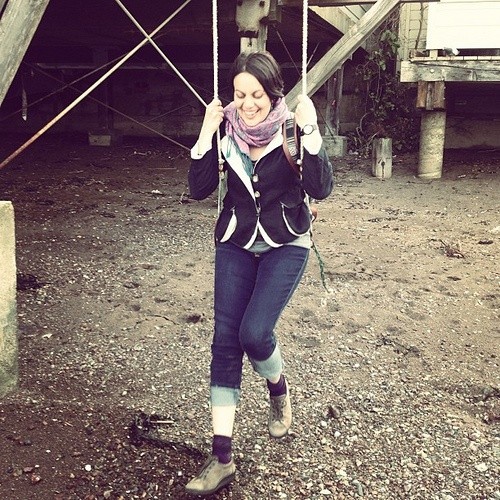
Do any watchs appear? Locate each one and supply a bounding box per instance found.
[298,123,319,136]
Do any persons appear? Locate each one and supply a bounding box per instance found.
[186,51,334,496]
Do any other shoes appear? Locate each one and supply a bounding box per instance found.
[270,378,292,438]
[186,455,236,493]
